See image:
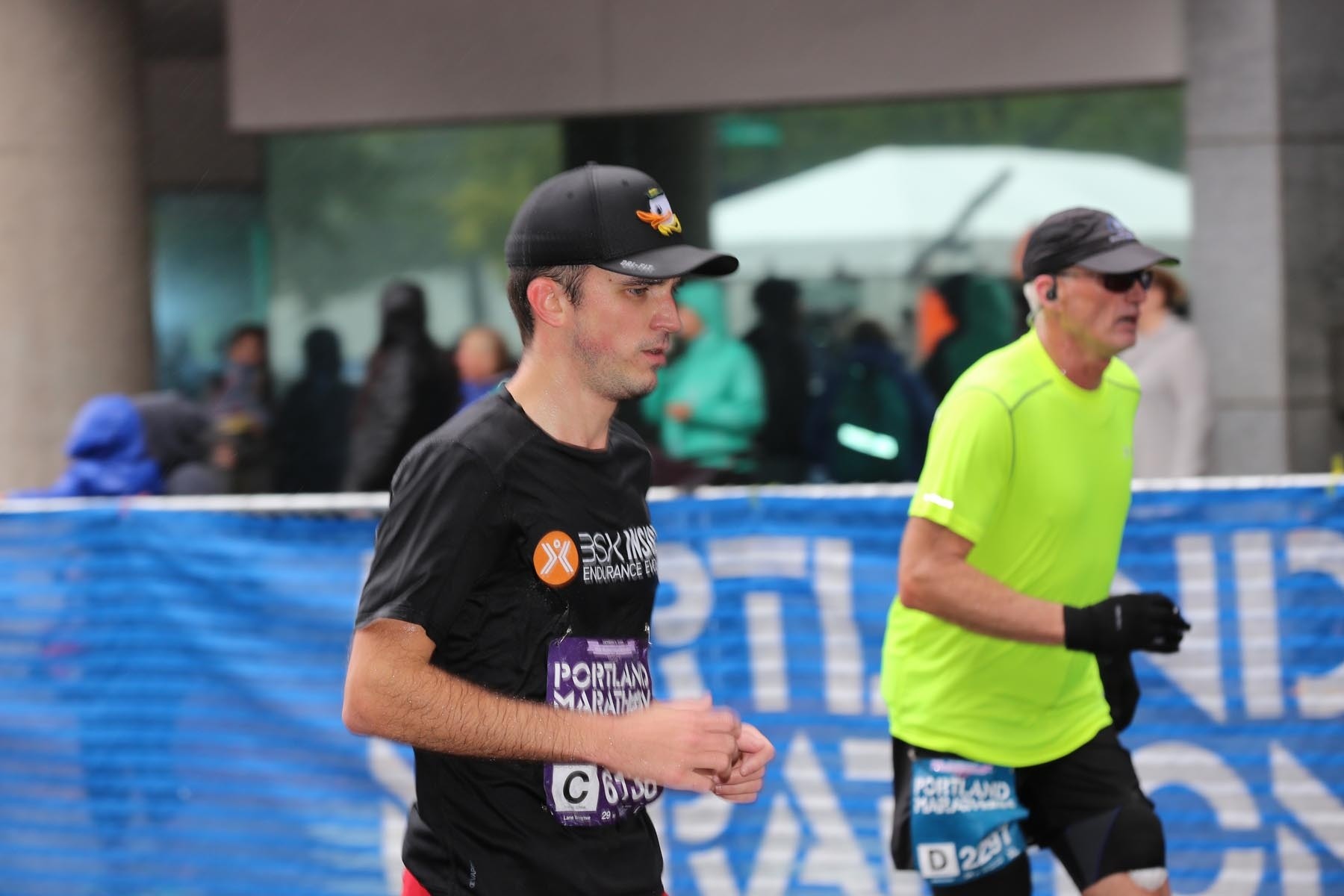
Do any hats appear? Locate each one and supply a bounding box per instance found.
[1021,208,1181,284]
[504,163,739,280]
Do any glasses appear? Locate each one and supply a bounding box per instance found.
[1051,270,1153,293]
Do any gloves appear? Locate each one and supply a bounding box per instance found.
[1063,592,1193,655]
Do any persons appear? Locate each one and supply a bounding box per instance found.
[881,207,1195,896]
[340,168,775,896]
[0,262,1215,504]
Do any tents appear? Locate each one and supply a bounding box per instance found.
[705,145,1191,340]
